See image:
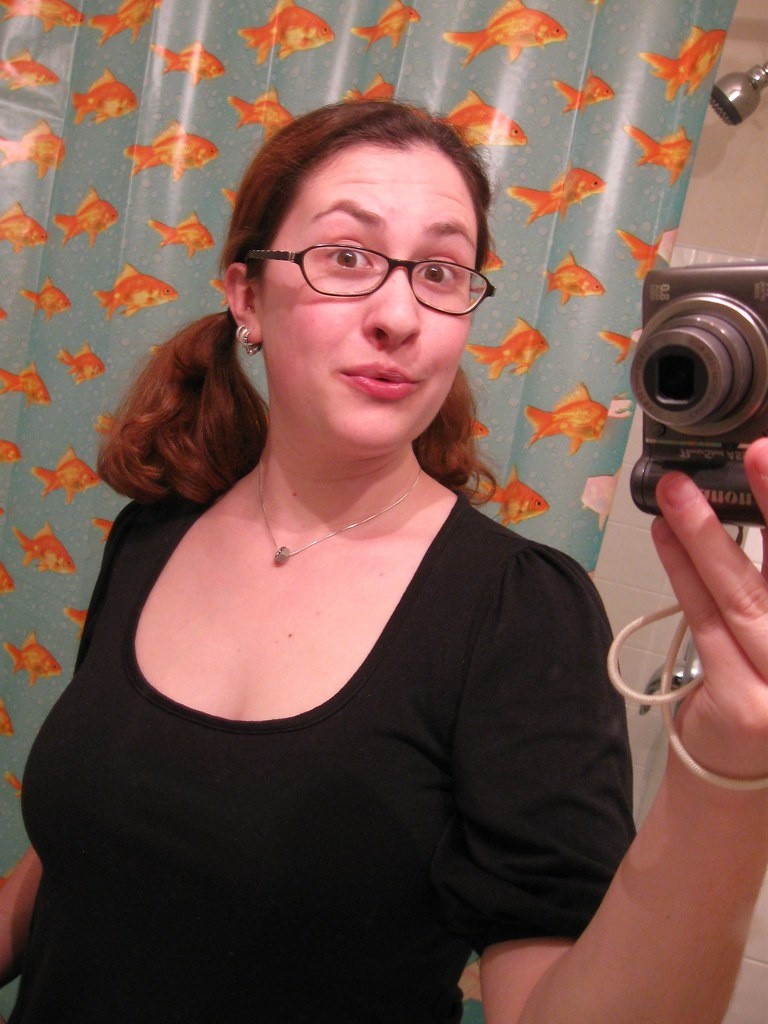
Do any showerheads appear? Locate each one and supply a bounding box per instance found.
[709,64,768,128]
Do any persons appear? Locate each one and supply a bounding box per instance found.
[0,98,768,1024]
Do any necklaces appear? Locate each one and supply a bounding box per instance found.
[251,440,423,566]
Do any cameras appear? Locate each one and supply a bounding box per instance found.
[629,259,768,527]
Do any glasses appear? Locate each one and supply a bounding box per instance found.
[247,245,496,316]
